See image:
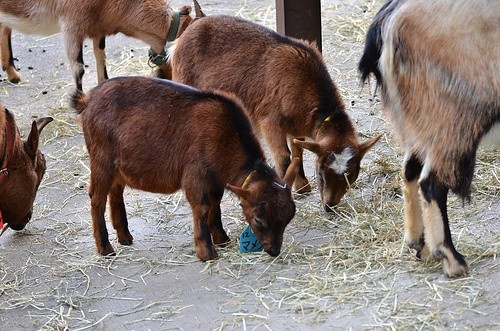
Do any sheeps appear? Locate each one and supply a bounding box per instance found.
[164,13,385,213]
[0,105,54,233]
[355,0,500,277]
[70,75,301,263]
[0,0,206,94]
